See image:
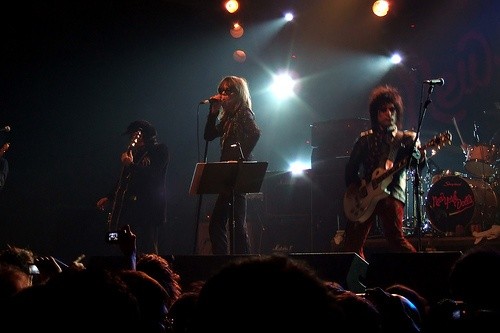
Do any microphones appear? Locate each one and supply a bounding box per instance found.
[200,99,209,104]
[423,77,445,87]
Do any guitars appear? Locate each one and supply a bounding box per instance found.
[343,131,452,224]
[108,127,143,233]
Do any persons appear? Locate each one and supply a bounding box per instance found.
[1,236,500,333]
[0,158,9,191]
[97,119,171,255]
[340,84,428,259]
[204,76,261,254]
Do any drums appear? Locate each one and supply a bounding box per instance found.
[464,142,500,179]
[426,175,498,236]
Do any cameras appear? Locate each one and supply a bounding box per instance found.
[27,263,40,275]
[105,230,125,244]
[452,308,468,319]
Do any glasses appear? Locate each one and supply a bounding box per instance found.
[218,87,234,95]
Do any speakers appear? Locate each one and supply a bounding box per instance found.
[159,250,463,284]
